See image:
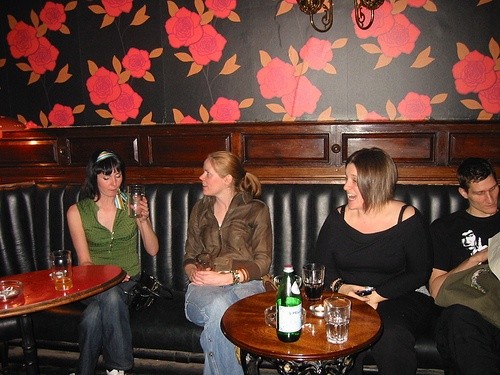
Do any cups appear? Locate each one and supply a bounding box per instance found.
[324,297,351,345]
[51,250,74,291]
[302,262,325,301]
[127,184,146,218]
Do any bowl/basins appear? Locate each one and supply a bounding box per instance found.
[265,305,306,328]
[49,270,68,279]
[274,275,302,291]
[309,304,324,317]
[0,280,23,302]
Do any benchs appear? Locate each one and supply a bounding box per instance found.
[0,183,500,375]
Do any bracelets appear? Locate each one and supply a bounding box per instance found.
[334,280,344,294]
[240,268,248,282]
[231,270,240,284]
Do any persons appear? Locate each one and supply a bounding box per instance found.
[422,157,500,375]
[314,147,432,375]
[66,151,159,375]
[185,151,272,375]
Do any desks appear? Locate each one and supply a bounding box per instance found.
[0,264,129,375]
[220,285,382,375]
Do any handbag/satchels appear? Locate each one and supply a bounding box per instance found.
[132,272,161,315]
[435,263,500,328]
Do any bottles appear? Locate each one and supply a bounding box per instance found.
[275,264,303,341]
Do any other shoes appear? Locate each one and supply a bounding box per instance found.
[106,368,125,375]
